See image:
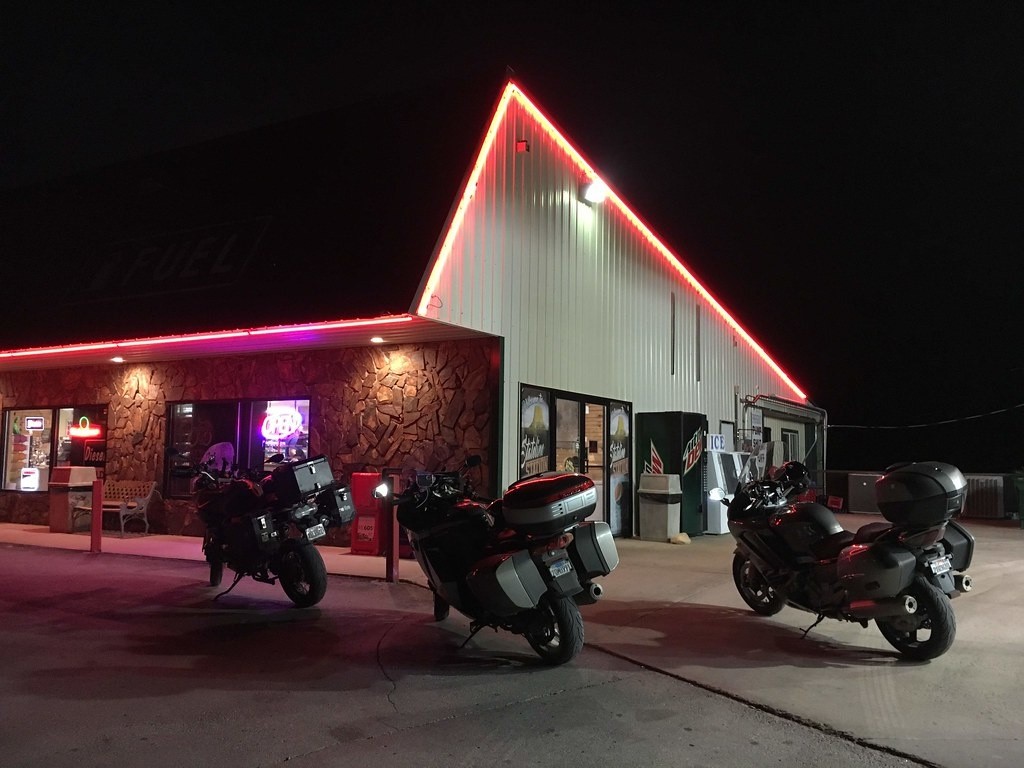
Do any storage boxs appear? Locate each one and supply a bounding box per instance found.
[318,484,357,526]
[569,521,621,576]
[838,539,911,599]
[875,463,970,529]
[943,521,974,575]
[274,454,334,498]
[230,511,278,553]
[500,472,596,532]
[476,549,547,613]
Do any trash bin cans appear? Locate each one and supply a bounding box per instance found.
[637,473,683,543]
[349,472,391,555]
[48,466,98,533]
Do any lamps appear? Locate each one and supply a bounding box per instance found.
[581,182,607,204]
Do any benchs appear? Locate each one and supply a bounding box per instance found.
[72,480,157,538]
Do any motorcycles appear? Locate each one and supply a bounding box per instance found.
[164,444,356,608]
[371,453,620,666]
[708,459,976,662]
